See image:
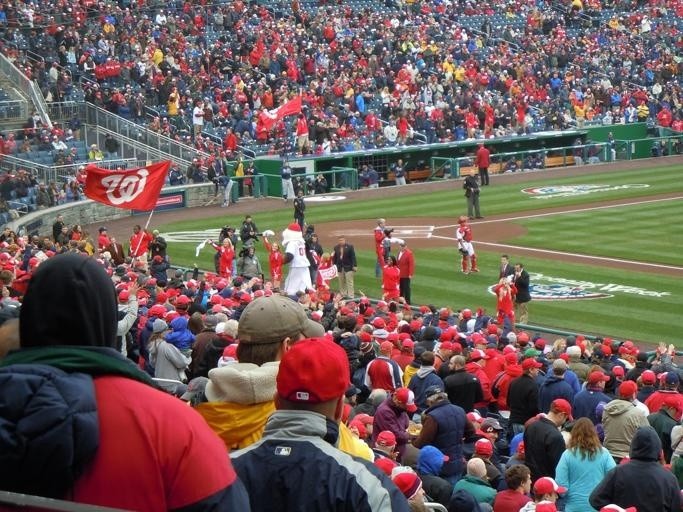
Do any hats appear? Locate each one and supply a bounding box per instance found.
[1,213,683,511]
[0,121,98,202]
[153,115,232,168]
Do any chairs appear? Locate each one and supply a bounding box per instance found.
[347,344,682,512]
[0,1,237,224]
[237,1,683,157]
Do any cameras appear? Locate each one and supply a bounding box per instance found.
[502,282,507,284]
